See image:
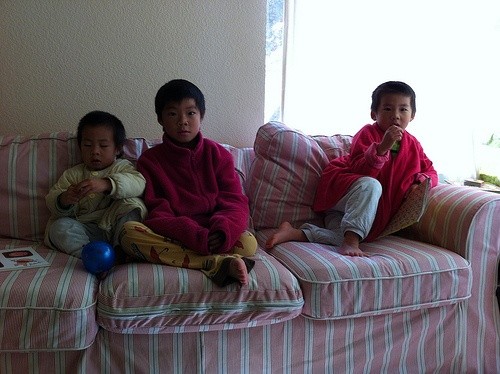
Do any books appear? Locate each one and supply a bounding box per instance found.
[464,179,484,187]
[0,246,51,272]
[375,178,431,239]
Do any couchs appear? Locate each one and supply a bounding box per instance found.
[0,121,500,374]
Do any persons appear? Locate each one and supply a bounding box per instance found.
[45,109,148,258]
[265,81,439,257]
[134,78,257,288]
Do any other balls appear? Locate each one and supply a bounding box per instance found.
[82,242,114,273]
